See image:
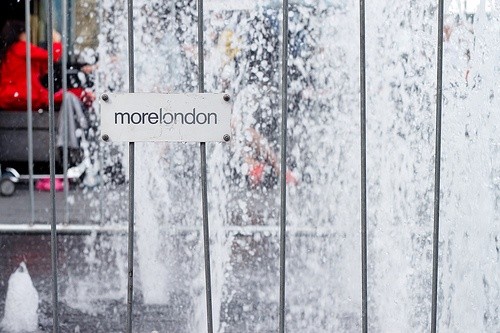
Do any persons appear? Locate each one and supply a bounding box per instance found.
[37,49,119,96]
[0,18,97,112]
